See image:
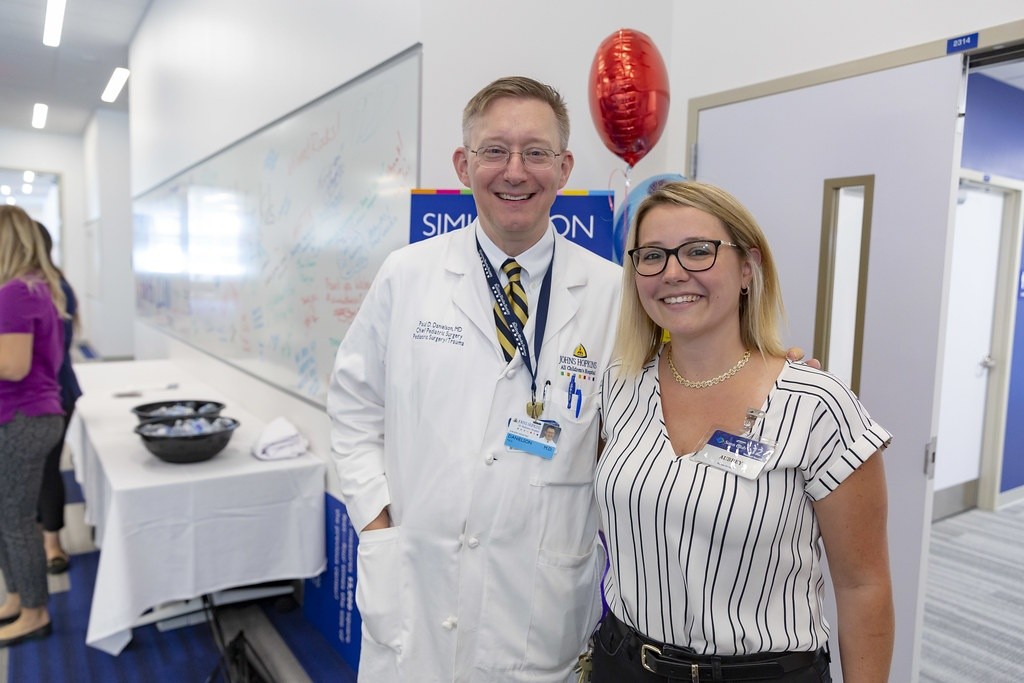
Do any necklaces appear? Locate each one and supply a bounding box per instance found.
[667,344,750,389]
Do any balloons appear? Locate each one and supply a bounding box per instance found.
[588,29,669,168]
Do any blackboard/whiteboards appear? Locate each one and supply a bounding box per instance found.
[130,43,421,412]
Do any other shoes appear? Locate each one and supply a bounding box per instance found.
[48,557,71,574]
[0,621,51,647]
[0,613,21,624]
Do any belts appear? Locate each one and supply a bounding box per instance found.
[606,611,823,681]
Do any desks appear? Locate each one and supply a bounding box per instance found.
[63,360,326,682]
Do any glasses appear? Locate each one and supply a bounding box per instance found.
[628,239,738,277]
[466,145,563,170]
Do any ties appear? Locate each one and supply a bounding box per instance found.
[493,259,528,364]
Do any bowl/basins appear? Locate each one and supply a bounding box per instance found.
[133,400,226,423]
[134,419,238,462]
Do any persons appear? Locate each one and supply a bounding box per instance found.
[0,203,74,647]
[539,425,556,446]
[586,181,896,683]
[327,75,822,683]
[27,220,83,573]
